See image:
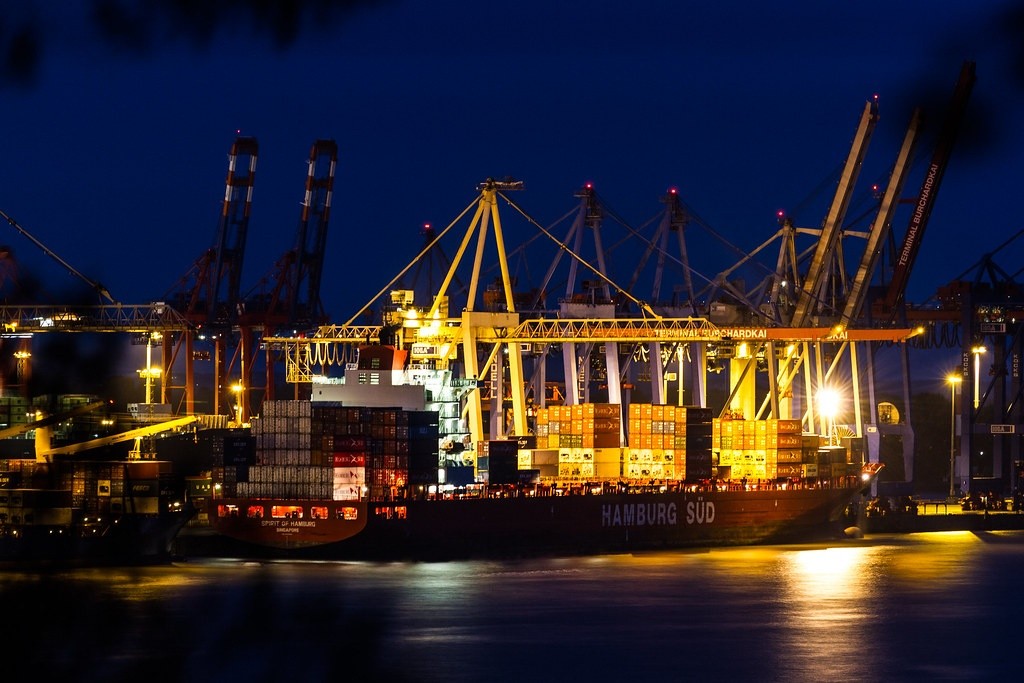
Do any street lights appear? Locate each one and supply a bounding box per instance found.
[942,366,964,500]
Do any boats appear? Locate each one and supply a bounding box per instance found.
[1,61,977,566]
